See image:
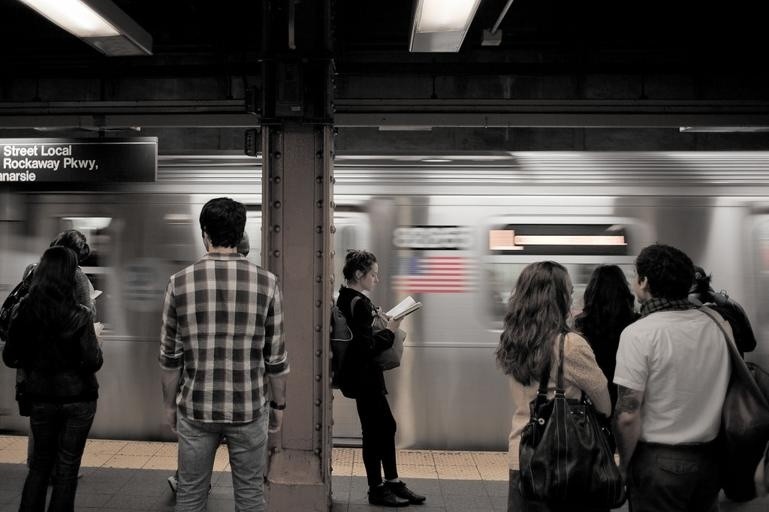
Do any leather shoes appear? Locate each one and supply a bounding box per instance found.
[369,480,426,506]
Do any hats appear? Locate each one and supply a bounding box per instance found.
[689,266,714,292]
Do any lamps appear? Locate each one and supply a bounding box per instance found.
[408,0,481,55]
[18,0,155,58]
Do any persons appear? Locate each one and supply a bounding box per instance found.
[158,198,291,511]
[493,260,612,512]
[0,228,102,512]
[337,249,426,508]
[612,243,757,512]
[164,232,250,496]
[570,264,640,453]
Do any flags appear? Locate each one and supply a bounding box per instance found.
[390,256,480,293]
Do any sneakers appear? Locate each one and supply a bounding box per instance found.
[167,476,178,492]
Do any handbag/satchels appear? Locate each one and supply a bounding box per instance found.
[721,362,768,500]
[518,396,626,510]
[331,305,350,355]
[371,306,407,370]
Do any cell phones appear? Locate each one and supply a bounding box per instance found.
[94,289,104,299]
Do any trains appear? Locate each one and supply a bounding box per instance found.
[0,146,769,451]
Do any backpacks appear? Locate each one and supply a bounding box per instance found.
[0,264,42,342]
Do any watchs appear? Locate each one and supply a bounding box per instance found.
[269,400,287,411]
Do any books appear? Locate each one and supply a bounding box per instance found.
[386,296,422,320]
